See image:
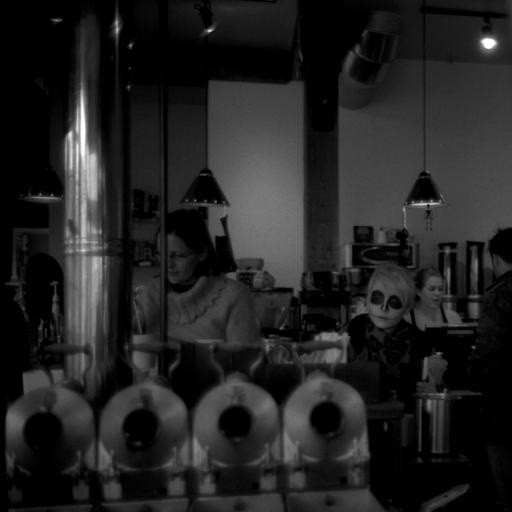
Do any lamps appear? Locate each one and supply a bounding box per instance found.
[406,1,443,209]
[181,0,231,208]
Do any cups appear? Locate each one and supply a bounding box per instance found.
[131,331,158,371]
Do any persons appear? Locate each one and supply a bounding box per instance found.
[121,208,261,345]
[333,263,427,414]
[397,266,456,329]
[465,224,510,491]
